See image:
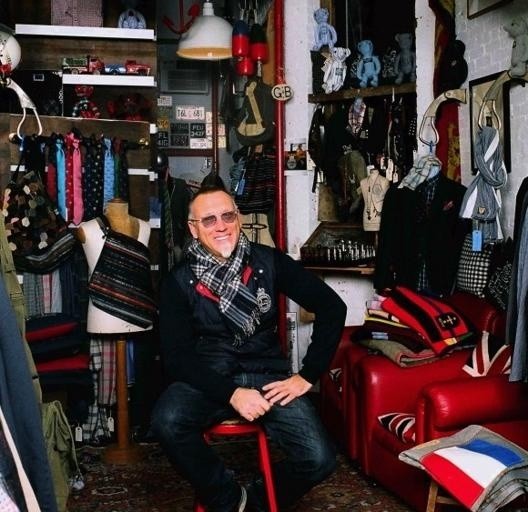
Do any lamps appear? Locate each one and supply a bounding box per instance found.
[174,0,235,62]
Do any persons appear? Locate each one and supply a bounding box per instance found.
[360,169,391,232]
[295,144,306,170]
[148,184,350,512]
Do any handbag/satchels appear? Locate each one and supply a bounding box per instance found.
[37,399,86,511]
[3,136,78,275]
[87,215,158,329]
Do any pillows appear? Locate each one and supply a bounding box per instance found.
[459,330,512,378]
[376,412,416,443]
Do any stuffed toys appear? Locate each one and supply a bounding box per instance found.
[310,8,416,95]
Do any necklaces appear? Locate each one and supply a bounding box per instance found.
[366,175,380,220]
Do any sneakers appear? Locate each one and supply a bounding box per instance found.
[205,478,247,512]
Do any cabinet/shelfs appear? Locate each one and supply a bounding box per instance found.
[9,24,158,174]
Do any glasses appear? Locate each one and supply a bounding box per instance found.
[188,208,238,229]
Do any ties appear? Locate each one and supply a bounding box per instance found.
[46,126,128,226]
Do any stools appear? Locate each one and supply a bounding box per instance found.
[426,479,461,512]
[196,418,279,512]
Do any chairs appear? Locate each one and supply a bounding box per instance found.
[362,313,528,512]
[319,290,497,461]
